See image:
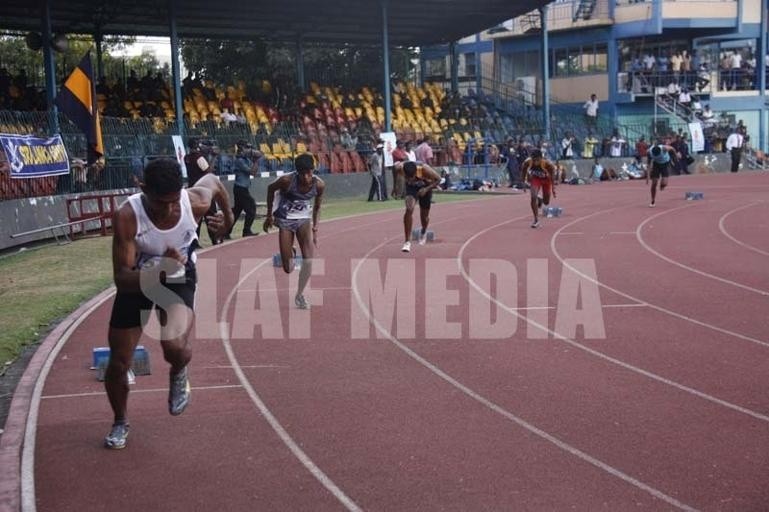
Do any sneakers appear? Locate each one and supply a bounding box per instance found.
[531,220,540,228]
[419,227,427,246]
[105,418,130,449]
[294,292,306,310]
[243,230,259,236]
[538,196,542,209]
[649,200,656,207]
[168,365,190,416]
[402,240,411,252]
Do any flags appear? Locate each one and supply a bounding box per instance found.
[51,49,105,155]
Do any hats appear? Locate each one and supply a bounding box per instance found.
[237,139,252,147]
[377,144,384,149]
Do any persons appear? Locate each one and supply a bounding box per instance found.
[183,133,225,247]
[262,149,325,308]
[341,47,769,254]
[0,68,47,110]
[225,139,261,243]
[98,70,248,132]
[102,156,236,449]
